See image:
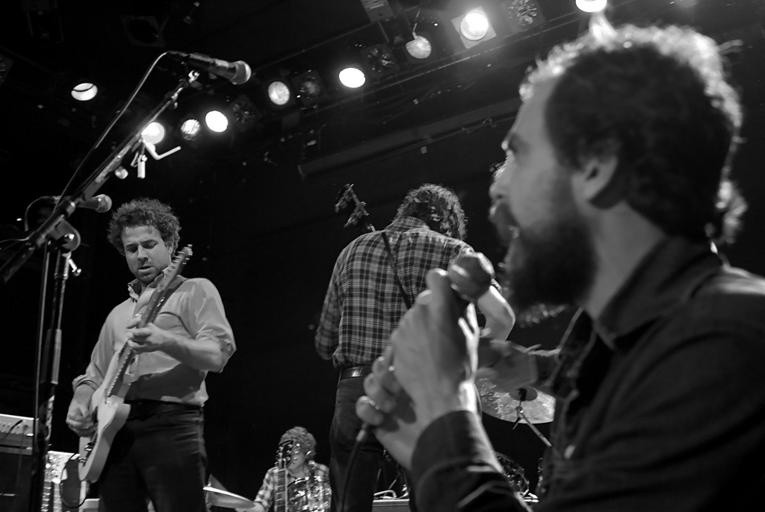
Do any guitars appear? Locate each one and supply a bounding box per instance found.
[274,440,300,511]
[78,243,193,483]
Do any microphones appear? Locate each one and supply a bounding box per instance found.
[360,253,493,429]
[167,50,251,88]
[55,193,112,214]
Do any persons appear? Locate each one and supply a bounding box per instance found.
[355,25,764,512]
[236,426,332,512]
[65,198,237,512]
[315,183,515,512]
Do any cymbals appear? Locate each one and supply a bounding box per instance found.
[475,378,555,423]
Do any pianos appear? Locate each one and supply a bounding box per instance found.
[0,414,34,456]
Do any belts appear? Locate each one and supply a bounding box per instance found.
[340,366,372,377]
[127,400,199,415]
